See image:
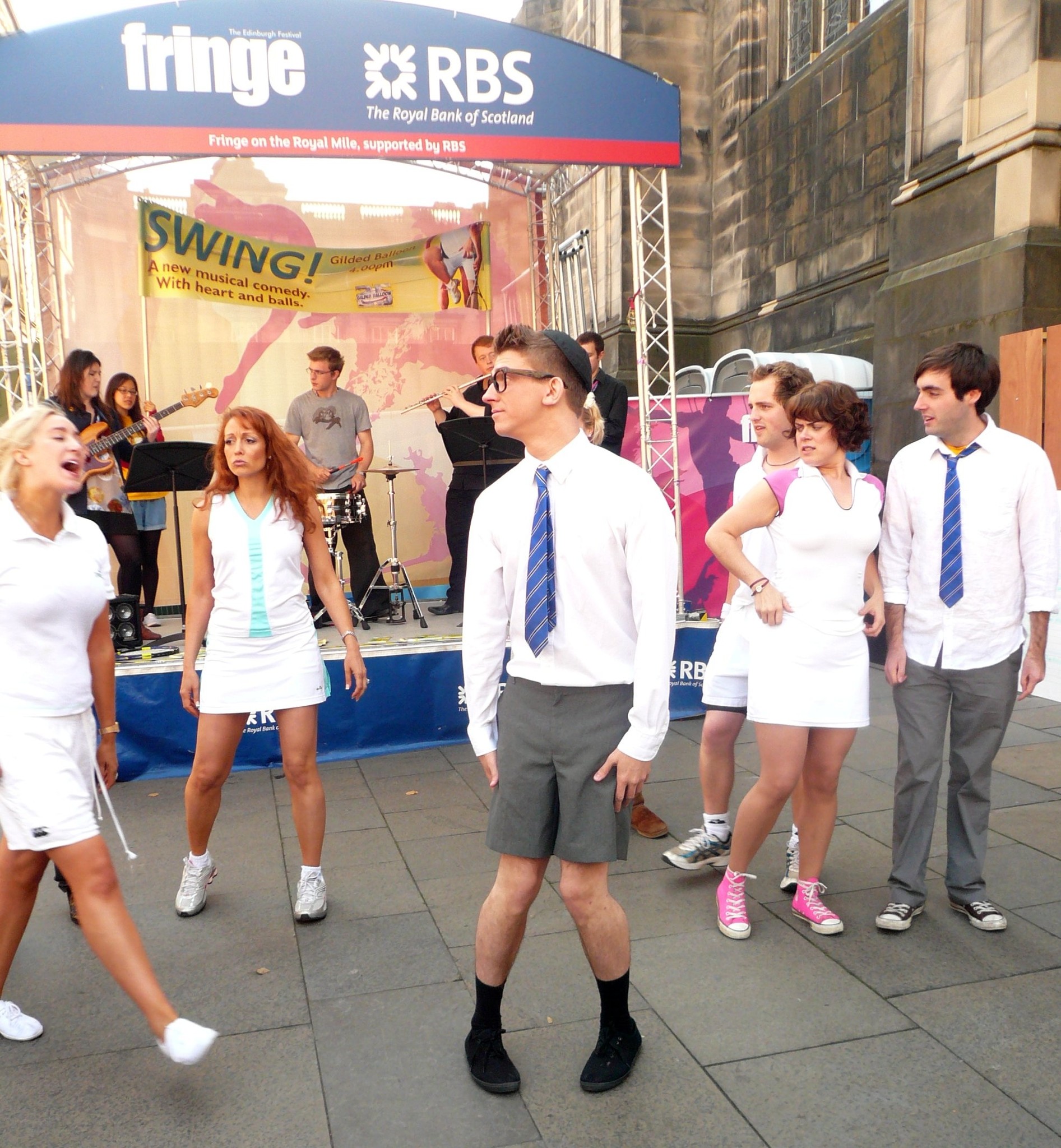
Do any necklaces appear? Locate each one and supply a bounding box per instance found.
[13,498,35,525]
[766,454,800,466]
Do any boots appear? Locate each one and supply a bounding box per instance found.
[139,608,161,639]
[630,792,668,838]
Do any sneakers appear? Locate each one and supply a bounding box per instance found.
[465,1028,521,1093]
[661,825,732,870]
[143,613,161,627]
[580,1016,642,1091]
[716,865,756,939]
[791,877,843,934]
[780,841,800,894]
[0,999,43,1041]
[295,870,327,922]
[175,856,218,916]
[447,278,461,303]
[876,902,925,930]
[159,1018,217,1064]
[949,897,1007,931]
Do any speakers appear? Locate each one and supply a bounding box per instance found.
[108,593,144,649]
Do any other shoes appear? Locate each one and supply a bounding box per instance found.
[66,890,80,923]
[376,609,389,617]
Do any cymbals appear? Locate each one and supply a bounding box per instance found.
[361,465,421,475]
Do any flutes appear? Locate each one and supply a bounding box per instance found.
[399,373,493,415]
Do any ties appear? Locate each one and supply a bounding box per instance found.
[938,442,980,608]
[524,468,556,659]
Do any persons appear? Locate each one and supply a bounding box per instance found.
[424,221,484,311]
[173,406,369,922]
[284,346,394,617]
[421,336,498,616]
[462,326,678,1093]
[575,330,628,455]
[631,340,1057,937]
[105,372,165,626]
[41,349,161,640]
[0,406,219,1065]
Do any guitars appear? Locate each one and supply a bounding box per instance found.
[67,388,217,479]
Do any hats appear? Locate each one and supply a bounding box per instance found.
[541,330,592,393]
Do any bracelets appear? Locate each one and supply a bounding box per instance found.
[341,630,358,644]
[750,577,767,589]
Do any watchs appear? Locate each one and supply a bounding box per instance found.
[751,580,769,596]
[98,721,120,735]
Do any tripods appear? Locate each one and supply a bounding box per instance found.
[308,473,428,630]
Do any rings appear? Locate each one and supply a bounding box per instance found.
[366,678,370,684]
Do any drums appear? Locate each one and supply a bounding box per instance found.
[315,492,367,525]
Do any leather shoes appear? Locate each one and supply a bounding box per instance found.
[428,604,458,614]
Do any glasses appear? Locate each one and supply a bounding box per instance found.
[118,388,138,395]
[306,367,333,376]
[488,367,567,393]
